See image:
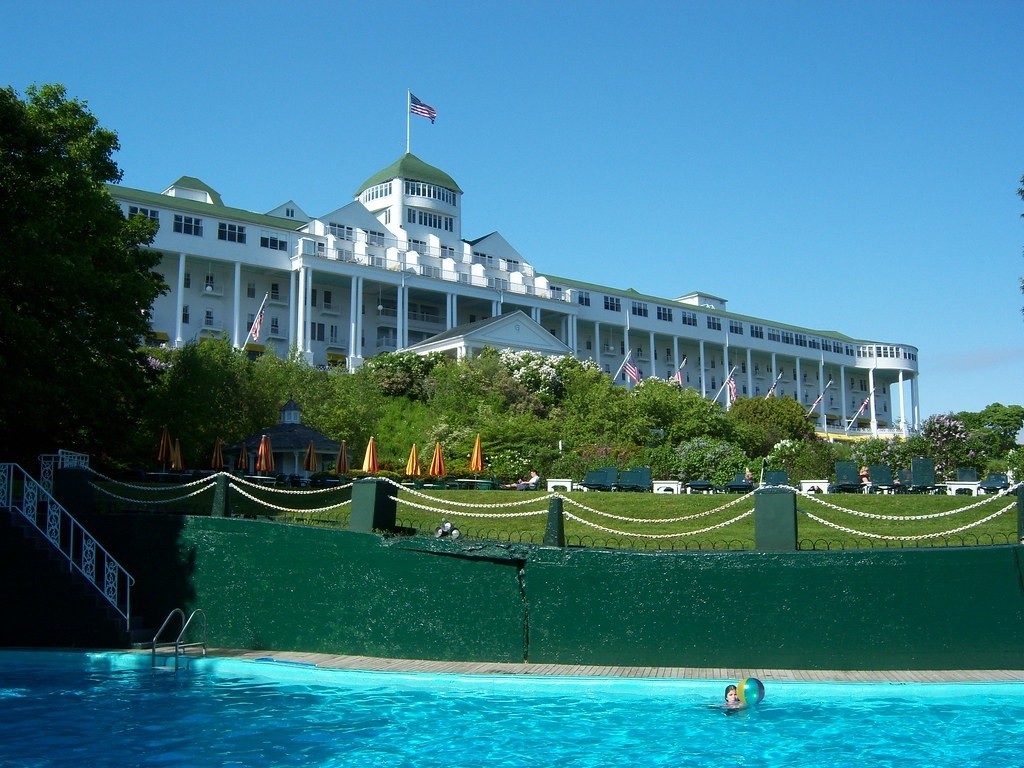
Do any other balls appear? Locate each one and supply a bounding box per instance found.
[736,677,765,704]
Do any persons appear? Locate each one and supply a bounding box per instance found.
[725,685,737,701]
[742,472,757,484]
[503,470,539,490]
[860,466,869,482]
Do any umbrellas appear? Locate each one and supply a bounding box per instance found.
[303,440,317,471]
[158,424,186,471]
[335,439,349,474]
[256,433,274,477]
[362,436,380,473]
[406,443,422,479]
[211,436,223,469]
[238,442,248,471]
[429,442,445,478]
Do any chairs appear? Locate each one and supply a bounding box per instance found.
[129,457,1009,496]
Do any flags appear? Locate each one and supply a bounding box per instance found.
[859,390,871,415]
[771,374,781,392]
[468,433,484,480]
[410,93,437,125]
[622,353,640,383]
[813,382,827,409]
[674,359,687,387]
[251,299,265,341]
[725,370,737,402]
